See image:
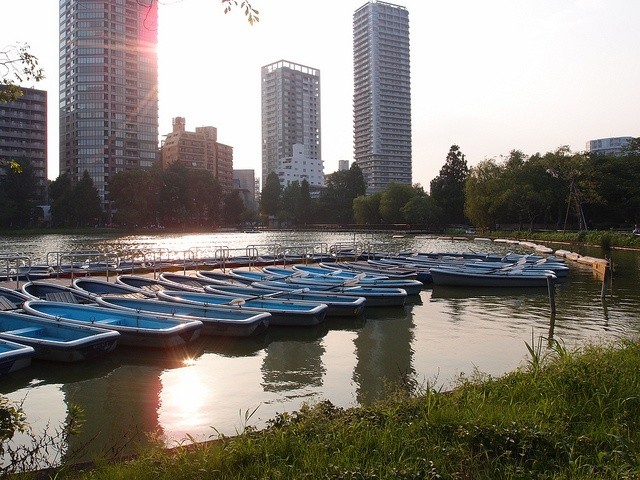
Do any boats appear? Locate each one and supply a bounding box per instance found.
[0,309,121,363]
[389,261,418,275]
[21,281,99,310]
[419,251,570,287]
[293,263,388,285]
[319,261,419,280]
[94,296,273,339]
[196,270,257,291]
[72,277,158,304]
[203,283,367,316]
[12,253,294,270]
[368,249,419,260]
[158,271,234,295]
[229,268,291,289]
[156,290,329,328]
[115,274,202,303]
[262,266,319,285]
[286,275,423,296]
[0,337,36,373]
[23,299,203,350]
[251,280,407,309]
[0,285,36,319]
[294,249,367,262]
[368,261,388,264]
